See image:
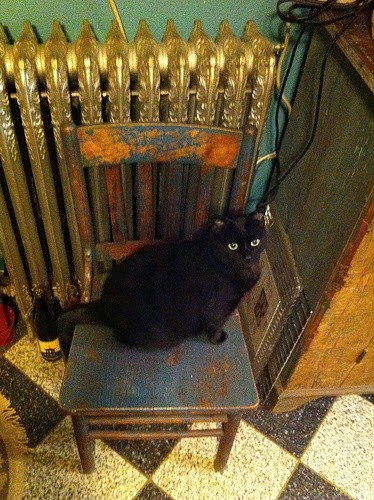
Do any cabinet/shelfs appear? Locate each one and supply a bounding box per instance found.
[236,2,374,416]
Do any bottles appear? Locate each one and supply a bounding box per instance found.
[34,290,62,361]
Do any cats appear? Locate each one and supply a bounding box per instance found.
[56,205,266,368]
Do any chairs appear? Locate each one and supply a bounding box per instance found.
[57,119,258,475]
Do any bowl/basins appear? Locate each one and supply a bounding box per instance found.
[0,306,15,348]
